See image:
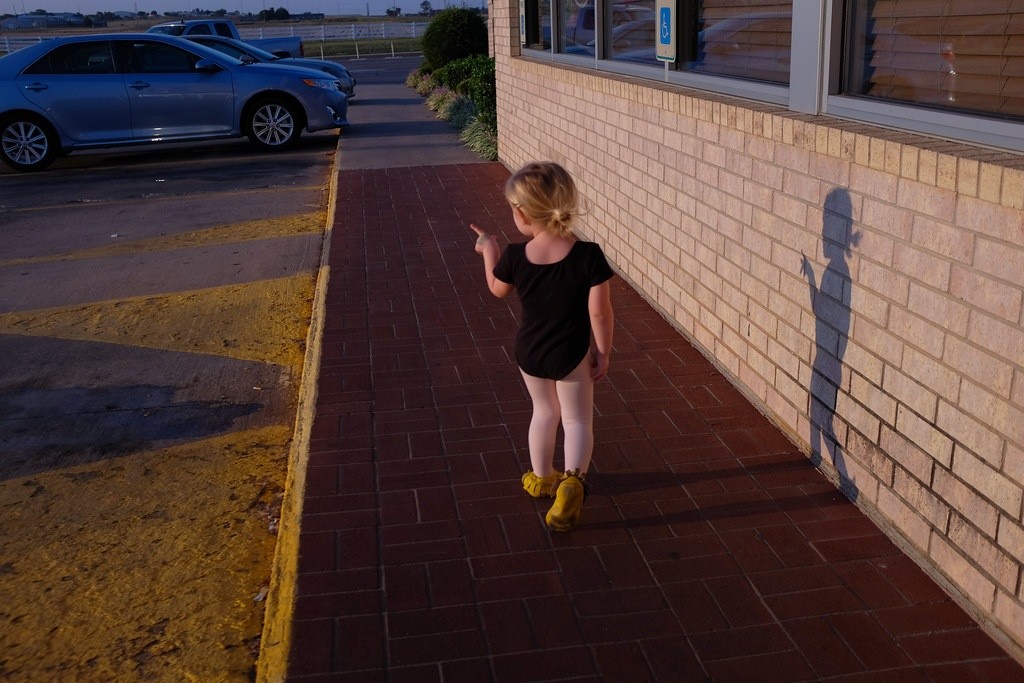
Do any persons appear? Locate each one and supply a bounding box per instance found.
[468,161,616,533]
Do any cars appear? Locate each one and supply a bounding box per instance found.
[0,32,350,173]
[133,33,356,99]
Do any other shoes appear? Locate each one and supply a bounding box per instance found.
[521,470,564,498]
[546,468,591,532]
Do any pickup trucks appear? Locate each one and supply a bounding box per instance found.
[87,18,304,68]
